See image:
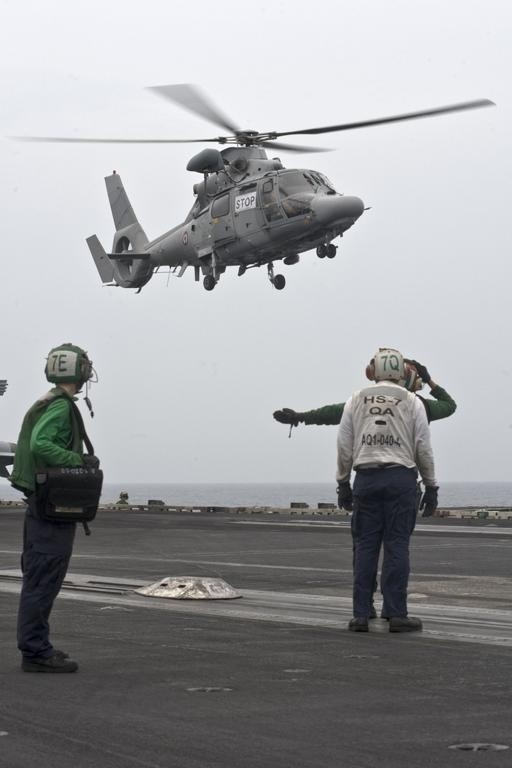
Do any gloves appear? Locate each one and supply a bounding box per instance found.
[273,408,299,428]
[420,485,439,517]
[338,481,353,510]
[82,452,101,471]
[405,357,431,383]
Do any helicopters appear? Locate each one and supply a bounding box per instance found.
[9,83,496,294]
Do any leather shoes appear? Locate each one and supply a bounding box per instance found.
[349,615,370,631]
[389,615,423,632]
[22,649,78,674]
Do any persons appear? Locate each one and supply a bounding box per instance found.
[336,348,438,632]
[8,344,103,674]
[273,359,457,619]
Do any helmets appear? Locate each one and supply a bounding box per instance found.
[366,349,423,391]
[46,343,88,383]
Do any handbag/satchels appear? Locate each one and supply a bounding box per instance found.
[35,467,101,522]
